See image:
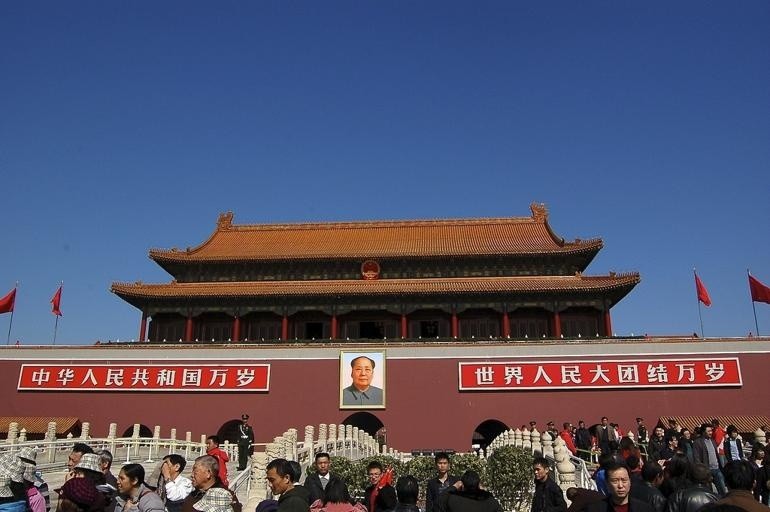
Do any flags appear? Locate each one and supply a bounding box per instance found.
[695,272,711,307]
[50,285,62,316]
[748,272,770,304]
[0,286,17,314]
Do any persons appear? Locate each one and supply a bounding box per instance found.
[2,412,770,512]
[342,354,384,406]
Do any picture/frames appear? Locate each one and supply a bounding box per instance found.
[339,348,386,409]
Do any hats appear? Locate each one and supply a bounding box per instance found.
[529,421,536,426]
[73,452,105,476]
[546,421,553,426]
[668,419,676,425]
[1,447,37,497]
[192,487,235,512]
[636,417,644,422]
[242,414,249,419]
